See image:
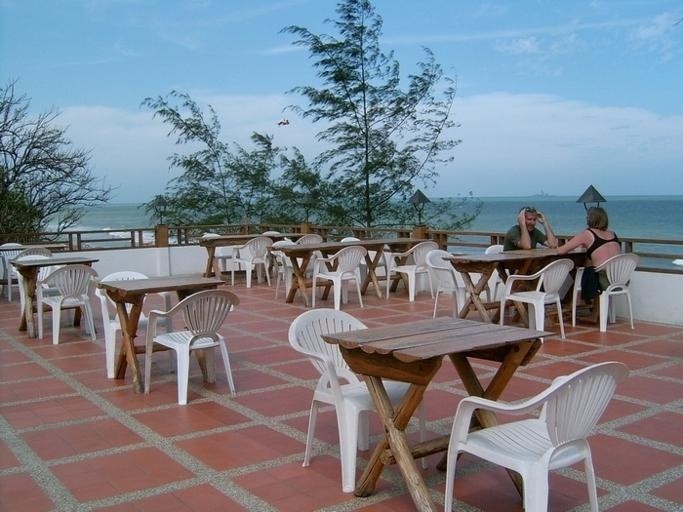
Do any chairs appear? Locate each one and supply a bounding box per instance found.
[0,230,640,511]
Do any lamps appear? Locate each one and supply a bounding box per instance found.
[407,188,432,229]
[575,183,608,211]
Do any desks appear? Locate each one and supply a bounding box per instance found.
[281,238,436,308]
[441,247,588,323]
[9,257,99,340]
[319,316,560,512]
[96,274,227,394]
[196,233,318,286]
[1,244,70,300]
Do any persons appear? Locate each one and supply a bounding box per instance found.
[556,207,623,325]
[496,205,557,292]
